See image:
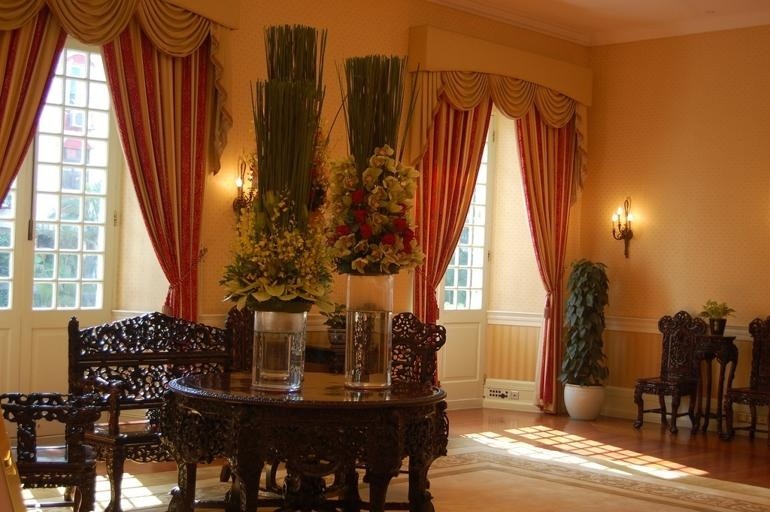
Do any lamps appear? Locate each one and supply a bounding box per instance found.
[611,206,633,258]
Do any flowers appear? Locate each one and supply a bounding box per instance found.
[218,23,338,311]
[317,55,427,274]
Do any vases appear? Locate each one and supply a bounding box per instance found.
[251,310,307,394]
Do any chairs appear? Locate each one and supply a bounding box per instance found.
[64,312,226,504]
[0,393,101,511]
[723,317,770,440]
[226,304,256,376]
[353,314,445,385]
[633,310,706,433]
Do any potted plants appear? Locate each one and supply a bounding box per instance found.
[319,299,345,345]
[701,301,734,335]
[557,257,608,421]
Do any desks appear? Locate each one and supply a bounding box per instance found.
[164,374,450,511]
[690,335,738,438]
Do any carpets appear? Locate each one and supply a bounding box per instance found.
[140,447,769,512]
